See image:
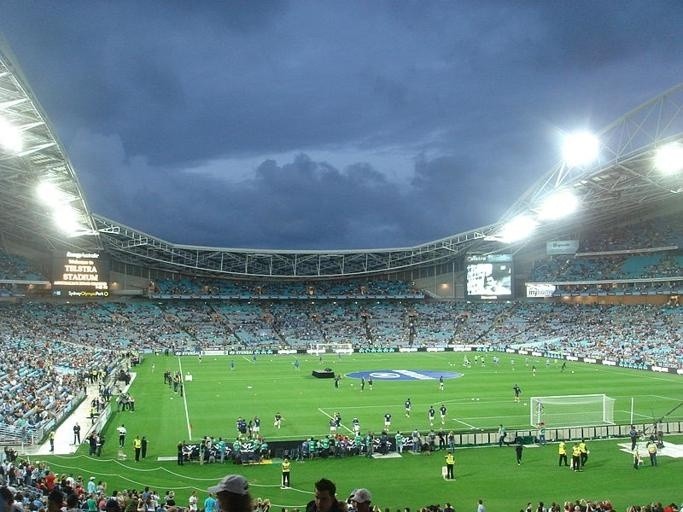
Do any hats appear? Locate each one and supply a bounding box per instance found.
[350,489,371,503]
[208,475,248,495]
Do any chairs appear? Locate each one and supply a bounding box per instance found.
[177,435,448,464]
[0,248,146,452]
[146,268,479,351]
[480,216,683,371]
